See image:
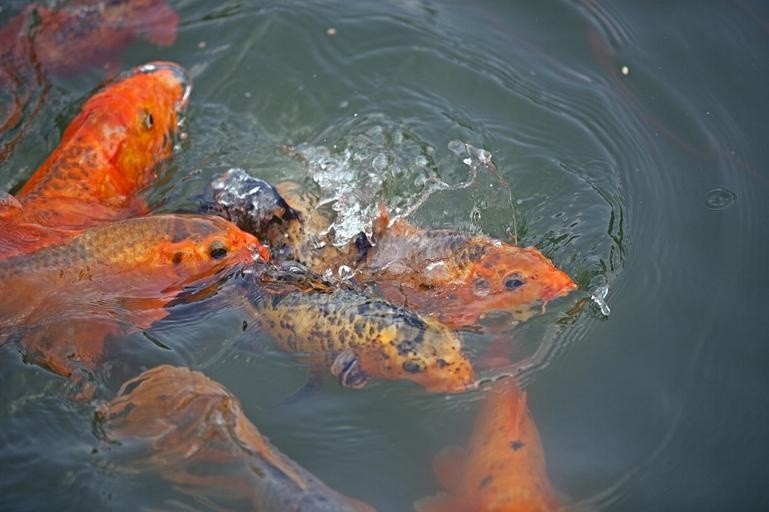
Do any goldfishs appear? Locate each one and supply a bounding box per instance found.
[0,0,582,512]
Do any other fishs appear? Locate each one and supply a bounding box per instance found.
[0,0,582,512]
